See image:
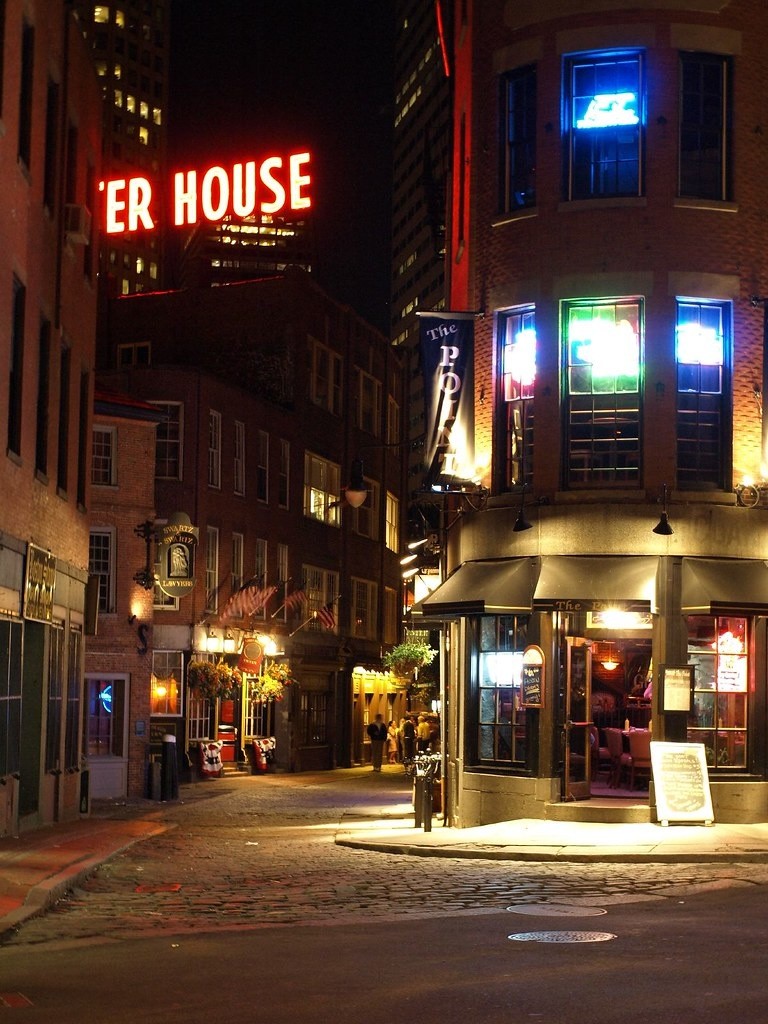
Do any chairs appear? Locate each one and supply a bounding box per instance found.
[590,726,653,792]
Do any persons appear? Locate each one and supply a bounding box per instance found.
[398,710,443,760]
[367,714,387,772]
[386,720,400,764]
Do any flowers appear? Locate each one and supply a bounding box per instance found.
[188,656,243,701]
[249,660,301,705]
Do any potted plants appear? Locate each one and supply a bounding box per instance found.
[383,642,439,676]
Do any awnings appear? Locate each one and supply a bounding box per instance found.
[399,558,768,630]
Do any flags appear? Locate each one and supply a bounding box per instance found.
[218,577,336,629]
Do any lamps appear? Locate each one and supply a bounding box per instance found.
[653,483,676,536]
[343,441,424,509]
[512,484,533,532]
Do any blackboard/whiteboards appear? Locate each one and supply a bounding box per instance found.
[648,740,714,822]
[657,663,696,716]
[520,663,546,709]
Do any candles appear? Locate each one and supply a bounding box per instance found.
[625,718,630,731]
[649,719,652,732]
